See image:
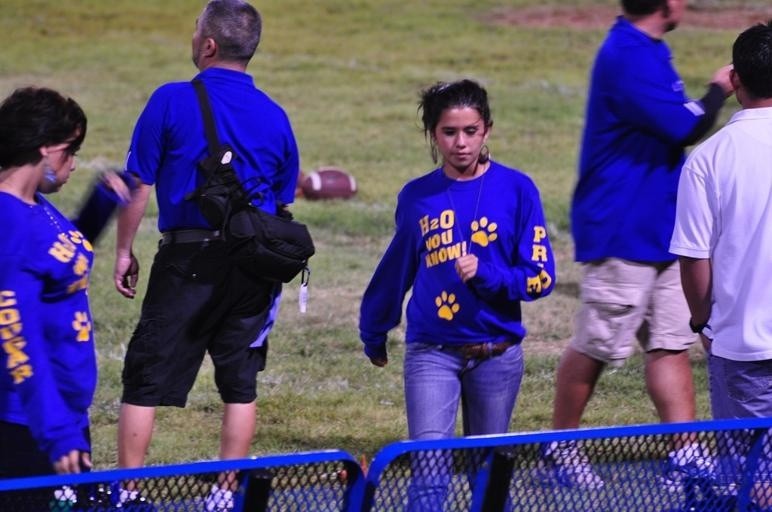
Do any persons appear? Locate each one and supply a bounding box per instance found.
[535,0,747,500]
[113,1,300,512]
[0,84,141,512]
[665,18,772,512]
[356,76,558,511]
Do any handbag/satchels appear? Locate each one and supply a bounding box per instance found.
[225,209,316,286]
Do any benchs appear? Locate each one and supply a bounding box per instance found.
[2,418,768,511]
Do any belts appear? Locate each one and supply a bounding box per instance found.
[440,342,514,360]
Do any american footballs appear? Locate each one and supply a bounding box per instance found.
[300,168,357,202]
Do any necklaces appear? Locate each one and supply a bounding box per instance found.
[441,159,486,257]
[44,207,61,233]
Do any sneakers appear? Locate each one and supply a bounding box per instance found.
[668,451,721,480]
[538,449,601,491]
